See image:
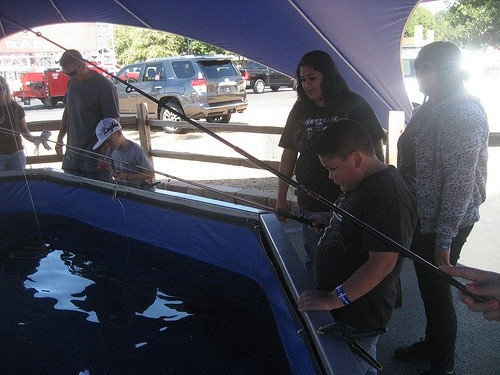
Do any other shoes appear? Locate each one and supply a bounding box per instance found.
[420,366,455,375]
[394,336,432,360]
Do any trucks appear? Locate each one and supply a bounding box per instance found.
[10,63,140,108]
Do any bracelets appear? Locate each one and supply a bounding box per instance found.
[335,285,351,305]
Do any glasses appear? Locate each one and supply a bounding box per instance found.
[62,63,83,76]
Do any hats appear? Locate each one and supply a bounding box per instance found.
[93,118,122,151]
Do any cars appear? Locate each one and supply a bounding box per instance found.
[231,59,299,93]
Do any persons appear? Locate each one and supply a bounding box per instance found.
[438,265,500,322]
[298,120,418,375]
[93,118,155,187]
[54,50,120,185]
[394,41,490,375]
[0,75,43,170]
[276,51,385,275]
[302,121,326,145]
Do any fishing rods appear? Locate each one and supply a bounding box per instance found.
[1,14,490,310]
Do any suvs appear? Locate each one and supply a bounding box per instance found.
[112,55,248,134]
